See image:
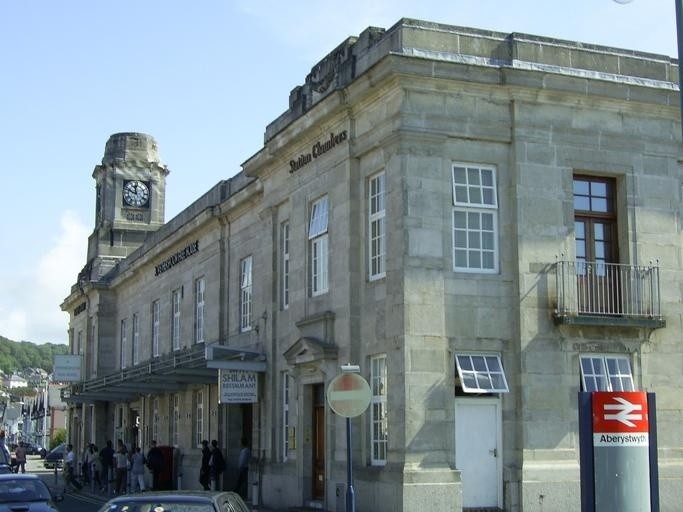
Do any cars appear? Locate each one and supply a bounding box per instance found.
[0,442,18,474]
[0,473,64,512]
[22,442,67,469]
[96,490,250,512]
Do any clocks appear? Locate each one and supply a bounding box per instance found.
[123,179,151,209]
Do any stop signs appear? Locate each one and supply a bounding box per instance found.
[326,372,372,418]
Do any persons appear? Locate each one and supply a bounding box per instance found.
[61,437,172,497]
[13,441,26,474]
[234,439,251,497]
[207,440,226,492]
[197,440,211,492]
